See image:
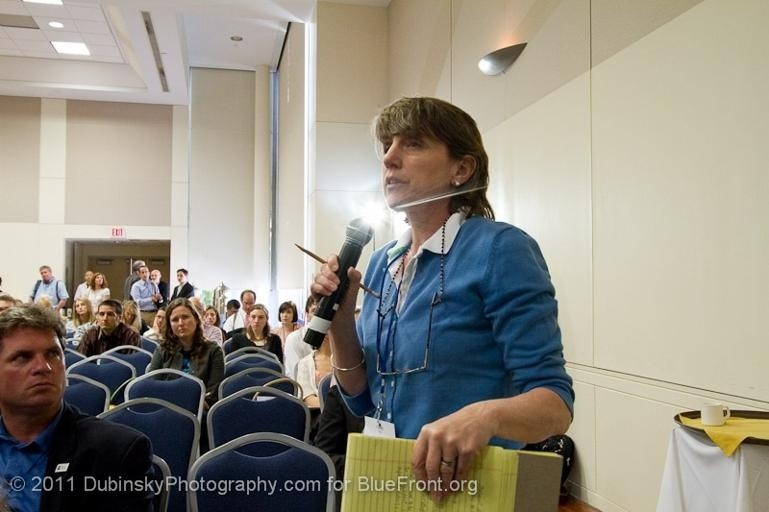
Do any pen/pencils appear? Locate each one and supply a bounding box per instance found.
[295,243,380,299]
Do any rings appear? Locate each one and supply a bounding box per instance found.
[440,458,455,466]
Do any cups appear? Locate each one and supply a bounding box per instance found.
[59,309,65,317]
[700,402,731,427]
[67,308,72,318]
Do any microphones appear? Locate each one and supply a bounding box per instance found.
[303,218,374,348]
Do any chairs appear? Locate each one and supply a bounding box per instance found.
[63,328,336,512]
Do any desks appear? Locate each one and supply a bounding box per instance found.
[656,424,769,512]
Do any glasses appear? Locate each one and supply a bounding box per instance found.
[375,291,439,377]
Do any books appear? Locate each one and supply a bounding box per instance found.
[337,432,563,512]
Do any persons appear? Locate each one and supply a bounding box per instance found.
[1,260,367,512]
[312,97,575,502]
